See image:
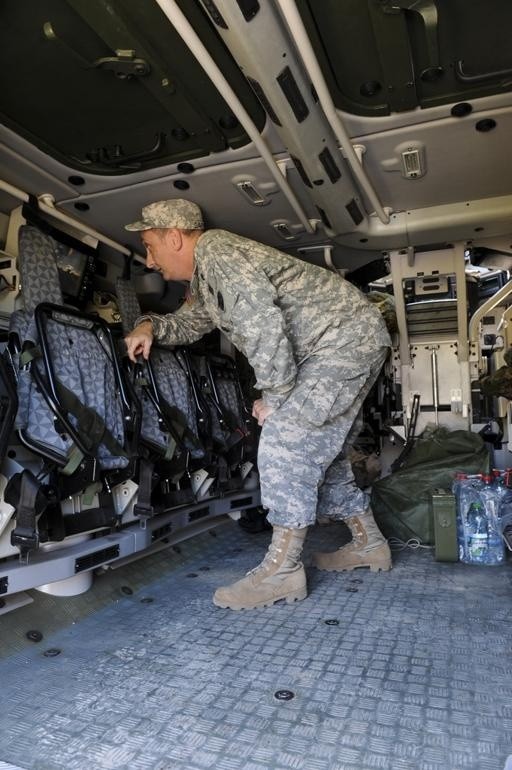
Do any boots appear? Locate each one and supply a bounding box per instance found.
[213,528,308,611]
[312,507,393,572]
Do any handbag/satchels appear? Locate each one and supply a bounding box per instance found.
[374,427,495,545]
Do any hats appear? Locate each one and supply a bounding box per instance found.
[124,198,203,232]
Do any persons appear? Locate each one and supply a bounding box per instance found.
[124,199,393,612]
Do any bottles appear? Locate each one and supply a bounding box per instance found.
[450,468,512,568]
